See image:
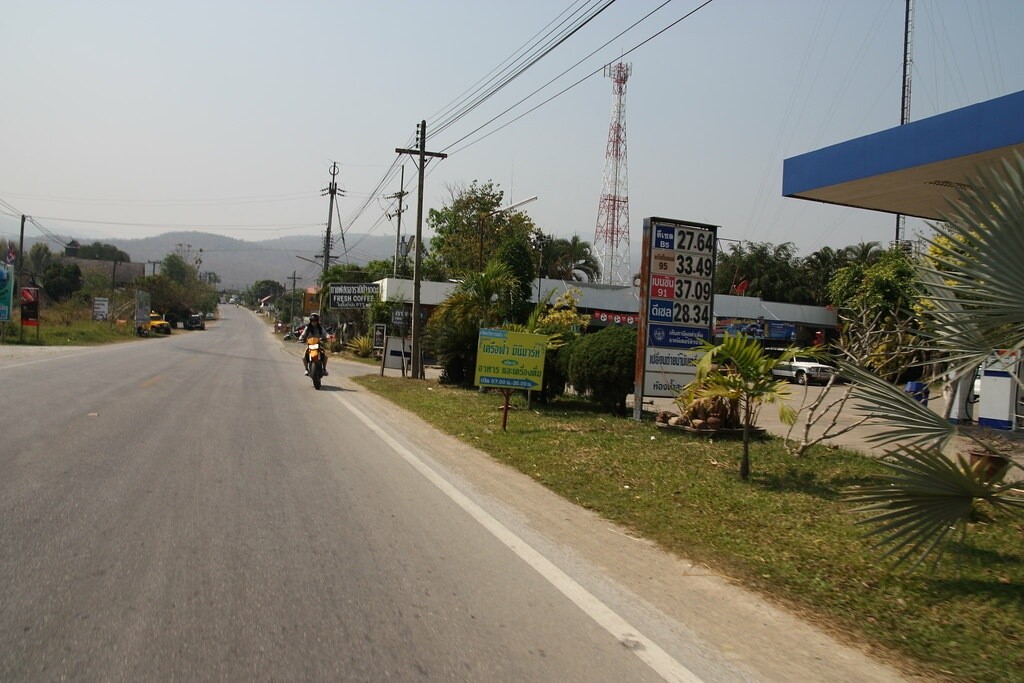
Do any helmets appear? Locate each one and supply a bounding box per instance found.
[309,313,319,320]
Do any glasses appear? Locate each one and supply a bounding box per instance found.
[310,319,318,322]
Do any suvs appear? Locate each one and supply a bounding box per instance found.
[763,346,837,386]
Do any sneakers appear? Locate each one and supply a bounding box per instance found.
[304,370,309,375]
[322,370,329,375]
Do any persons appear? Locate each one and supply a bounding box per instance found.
[137,324,147,335]
[299,314,330,375]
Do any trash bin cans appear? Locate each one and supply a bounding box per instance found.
[904,382,930,408]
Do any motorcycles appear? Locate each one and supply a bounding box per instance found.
[300,337,328,389]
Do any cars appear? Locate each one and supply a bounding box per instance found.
[205,313,215,320]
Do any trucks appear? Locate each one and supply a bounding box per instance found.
[115,312,172,335]
[186,314,206,331]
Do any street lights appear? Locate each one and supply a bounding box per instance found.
[479,195,538,275]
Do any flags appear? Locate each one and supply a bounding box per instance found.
[8,240,16,262]
